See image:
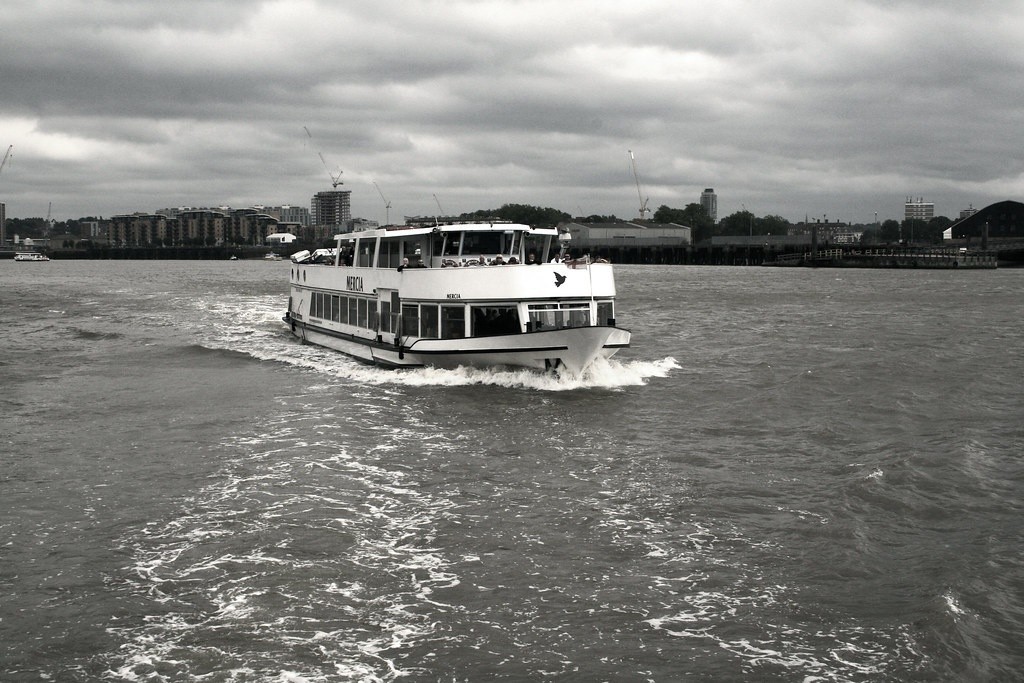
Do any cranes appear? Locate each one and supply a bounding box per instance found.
[373,181,393,224]
[432,193,447,216]
[302,126,343,189]
[628,149,652,218]
[0,145,14,172]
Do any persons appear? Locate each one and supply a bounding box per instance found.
[413,259,427,268]
[477,251,609,267]
[397,257,413,273]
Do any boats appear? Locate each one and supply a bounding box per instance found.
[229,255,237,260]
[13,252,50,261]
[264,251,281,262]
[282,220,633,373]
